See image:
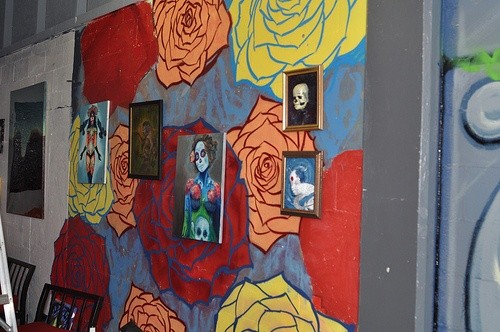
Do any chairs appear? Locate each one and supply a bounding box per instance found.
[0,257,36,325]
[33,282,105,332]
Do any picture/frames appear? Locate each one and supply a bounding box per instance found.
[127,98,163,180]
[282,65,324,132]
[280,150,324,218]
